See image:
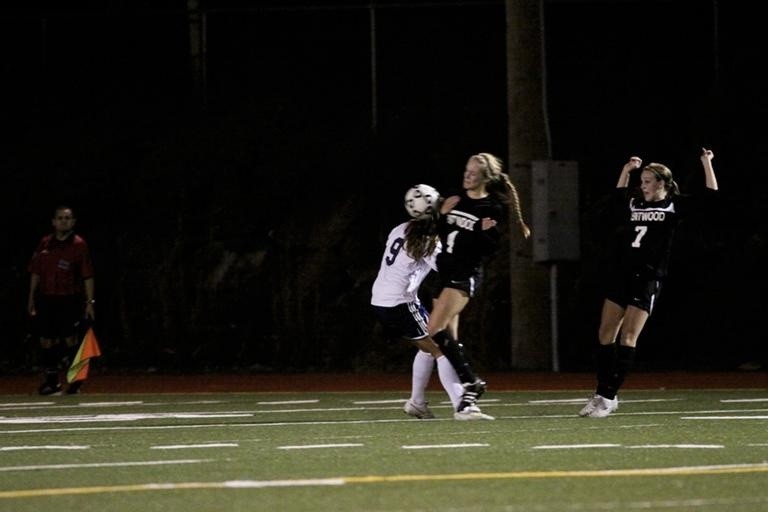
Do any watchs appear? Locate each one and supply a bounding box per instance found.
[86,297,96,305]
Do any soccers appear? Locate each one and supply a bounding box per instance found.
[404,183,444,220]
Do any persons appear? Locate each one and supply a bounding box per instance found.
[577,147,721,417]
[425,150,532,413]
[370,184,496,422]
[27,205,97,396]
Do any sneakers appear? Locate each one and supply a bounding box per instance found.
[404,401,435,419]
[38,380,80,396]
[579,394,618,419]
[454,380,495,421]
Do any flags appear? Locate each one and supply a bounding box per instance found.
[66,327,102,385]
